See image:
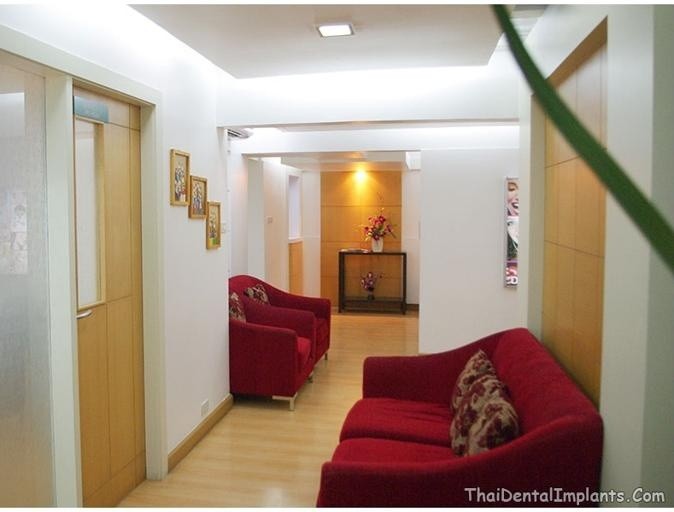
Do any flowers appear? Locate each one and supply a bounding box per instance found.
[358,208,397,243]
[360,271,383,292]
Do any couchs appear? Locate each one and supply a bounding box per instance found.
[229,274,331,364]
[316,328,604,507]
[229,279,317,411]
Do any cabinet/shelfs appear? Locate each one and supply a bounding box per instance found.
[338,251,407,315]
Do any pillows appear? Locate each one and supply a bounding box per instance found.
[243,283,271,305]
[450,374,508,456]
[450,349,497,418]
[229,291,246,322]
[463,389,520,457]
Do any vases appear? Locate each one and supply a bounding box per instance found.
[368,287,375,300]
[371,237,383,253]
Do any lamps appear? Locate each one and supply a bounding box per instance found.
[317,23,354,37]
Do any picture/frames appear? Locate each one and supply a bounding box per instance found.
[169,148,191,207]
[503,176,519,288]
[188,175,208,220]
[206,201,222,251]
